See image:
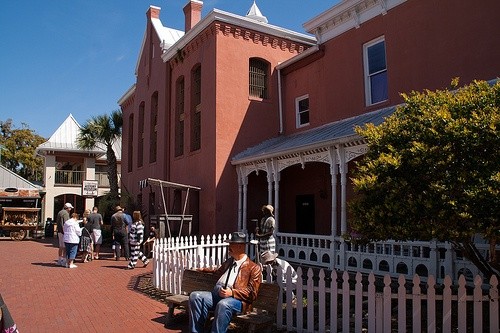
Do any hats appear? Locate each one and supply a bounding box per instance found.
[261,250,278,264]
[115,206,122,211]
[224,232,249,244]
[64,203,74,209]
[262,205,274,216]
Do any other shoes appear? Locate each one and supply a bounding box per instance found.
[56,261,65,266]
[143,262,149,268]
[65,263,69,268]
[125,267,134,270]
[68,264,77,268]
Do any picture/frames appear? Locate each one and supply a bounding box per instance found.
[82,180,98,196]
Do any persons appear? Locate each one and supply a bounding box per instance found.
[260,246,299,303]
[55,203,104,268]
[188,232,262,333]
[254,204,276,259]
[141,222,160,258]
[125,211,150,270]
[111,205,132,260]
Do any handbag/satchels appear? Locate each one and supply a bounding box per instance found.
[126,227,128,232]
[90,237,92,243]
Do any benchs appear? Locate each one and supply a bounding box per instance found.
[166,269,280,333]
